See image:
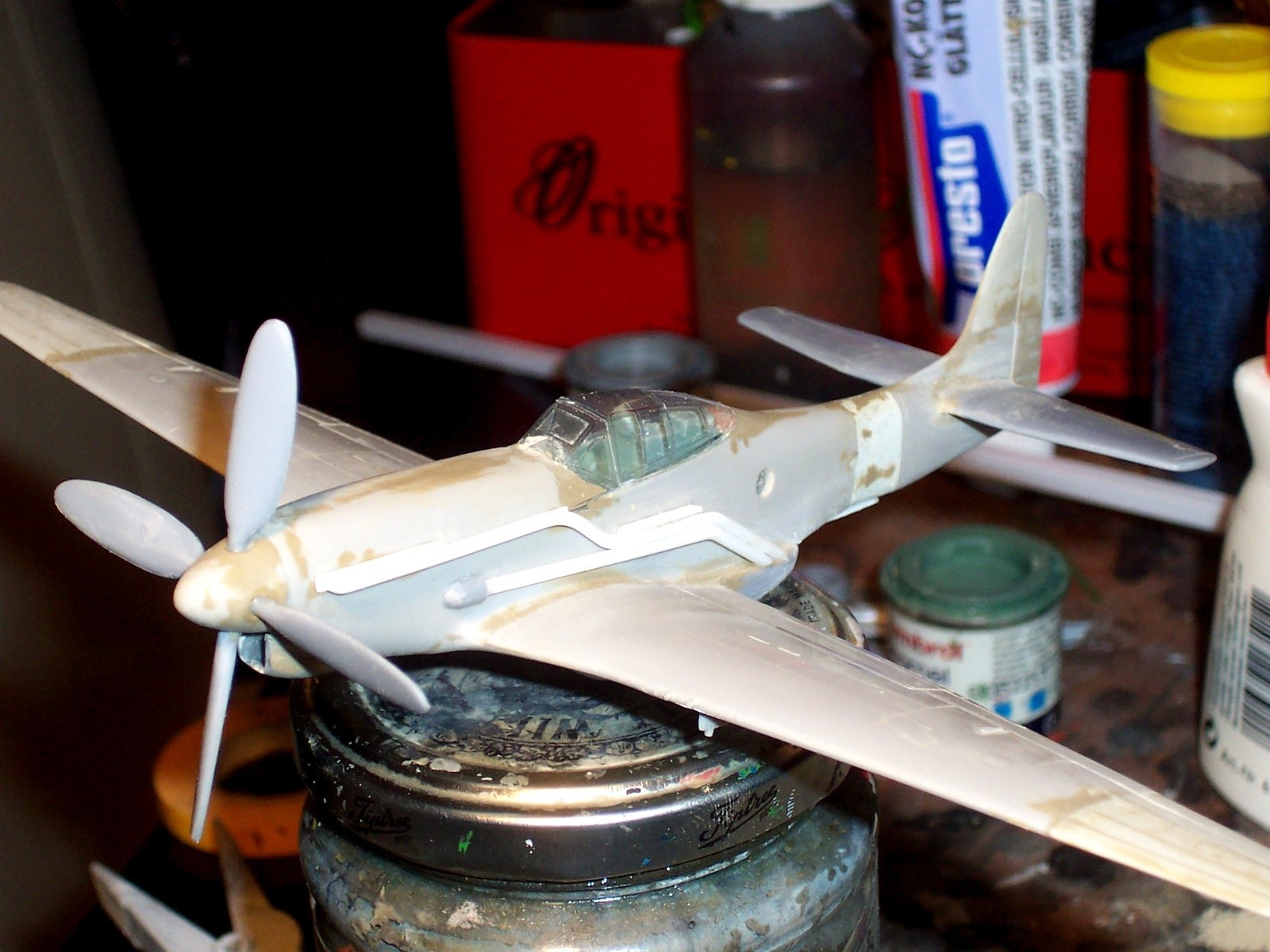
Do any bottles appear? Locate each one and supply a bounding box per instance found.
[295,572,878,952]
[1197,301,1269,836]
[1144,23,1270,494]
[681,0,884,397]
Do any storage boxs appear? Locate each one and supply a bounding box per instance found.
[449,0,703,346]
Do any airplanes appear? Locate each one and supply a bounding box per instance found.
[1,192,1270,921]
